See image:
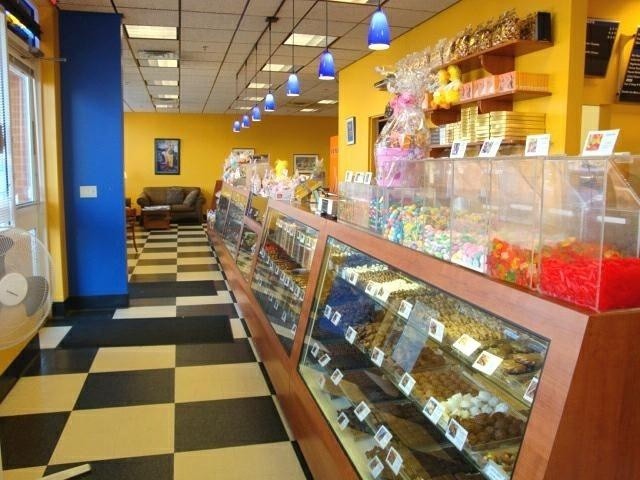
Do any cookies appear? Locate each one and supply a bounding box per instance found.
[334,259,545,383]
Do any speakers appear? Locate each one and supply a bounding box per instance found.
[535,12,551,42]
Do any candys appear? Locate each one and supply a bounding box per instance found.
[488,239,542,290]
[450,243,487,274]
[424,225,453,263]
[368,199,449,252]
[540,248,640,312]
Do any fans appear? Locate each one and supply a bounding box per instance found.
[0,227,56,350]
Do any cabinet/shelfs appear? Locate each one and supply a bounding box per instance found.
[416,39,552,149]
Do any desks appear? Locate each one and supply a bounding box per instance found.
[137,186,207,225]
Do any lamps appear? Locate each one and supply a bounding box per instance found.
[233,76,241,133]
[251,44,261,121]
[318,0,336,81]
[264,22,275,112]
[367,0,391,50]
[241,62,250,129]
[286,0,300,96]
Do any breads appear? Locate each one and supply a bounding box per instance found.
[302,338,483,480]
[327,302,524,472]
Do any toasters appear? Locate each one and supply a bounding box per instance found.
[318,196,338,219]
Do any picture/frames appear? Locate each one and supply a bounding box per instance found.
[153,138,180,176]
[293,154,319,174]
[346,117,355,145]
